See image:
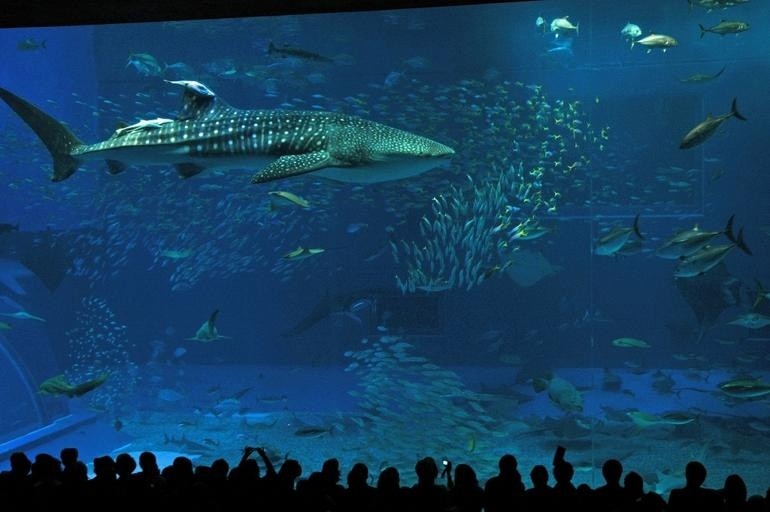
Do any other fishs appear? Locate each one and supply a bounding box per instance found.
[0,0,770,497]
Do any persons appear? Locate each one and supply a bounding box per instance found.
[0,443,770,511]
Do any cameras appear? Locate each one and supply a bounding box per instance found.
[443,460,449,467]
[250,447,260,451]
